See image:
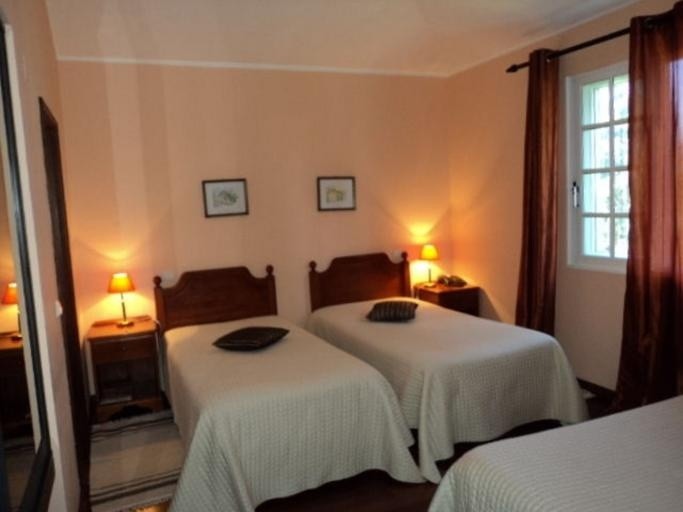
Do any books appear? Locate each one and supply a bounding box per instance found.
[98,384,133,407]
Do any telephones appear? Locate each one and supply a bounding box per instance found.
[438,274,467,287]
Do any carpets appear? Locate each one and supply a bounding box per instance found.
[89,409,194,509]
[0,431,35,509]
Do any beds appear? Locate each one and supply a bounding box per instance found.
[306,250,602,484]
[151,263,428,511]
[422,395,681,511]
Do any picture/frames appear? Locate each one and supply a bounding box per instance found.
[316,176,358,211]
[201,178,250,218]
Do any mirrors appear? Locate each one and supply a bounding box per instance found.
[0,26,59,512]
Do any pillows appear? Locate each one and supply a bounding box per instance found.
[365,298,418,325]
[212,318,290,354]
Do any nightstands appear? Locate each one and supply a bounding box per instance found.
[413,280,481,317]
[0,334,28,443]
[85,315,165,423]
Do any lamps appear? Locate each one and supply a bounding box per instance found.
[0,282,20,346]
[108,271,137,327]
[419,244,440,290]
